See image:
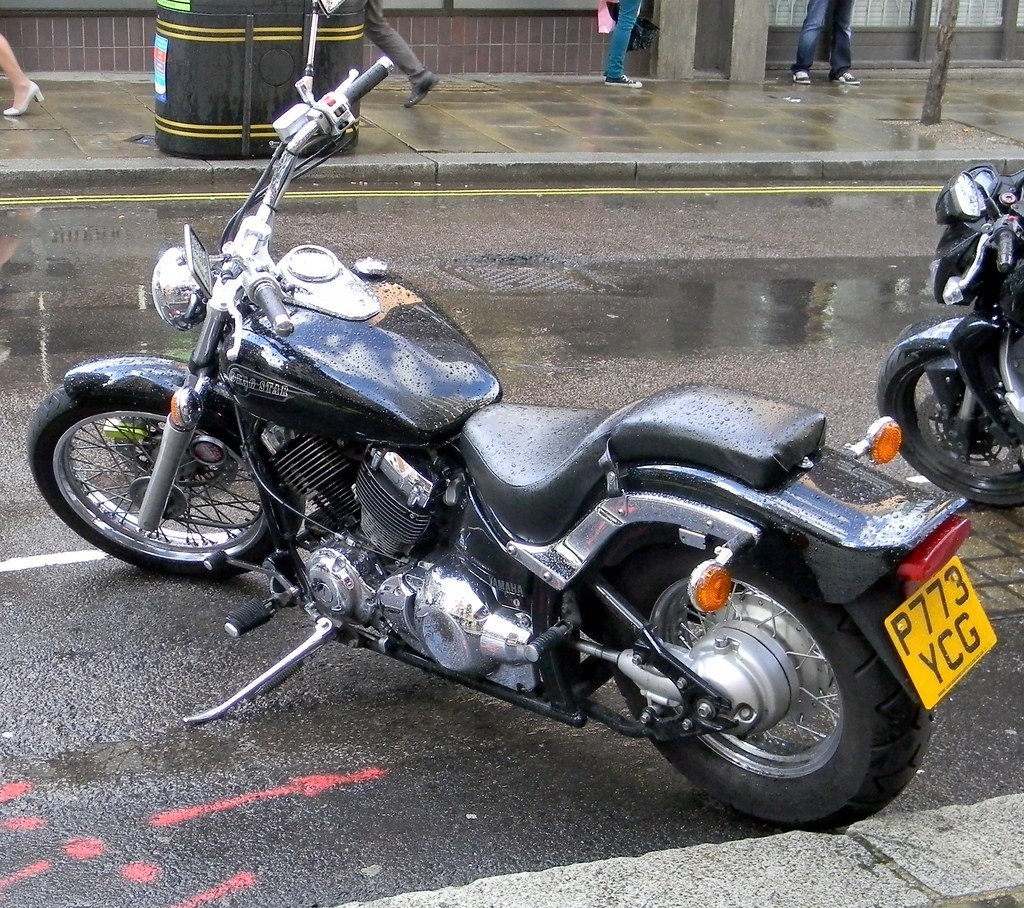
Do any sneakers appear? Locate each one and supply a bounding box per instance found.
[603,74,643,89]
[792,71,811,83]
[602,73,607,81]
[831,71,861,84]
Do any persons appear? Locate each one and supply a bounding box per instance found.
[0,33,44,115]
[791,0,860,84]
[598,0,643,88]
[366,0,438,108]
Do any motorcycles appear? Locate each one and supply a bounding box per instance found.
[875,163,1024,506]
[27,0,998,831]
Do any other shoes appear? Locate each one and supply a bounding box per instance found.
[404,72,440,107]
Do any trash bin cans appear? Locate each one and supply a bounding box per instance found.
[155,0,367,159]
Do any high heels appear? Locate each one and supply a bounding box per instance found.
[3,81,45,116]
